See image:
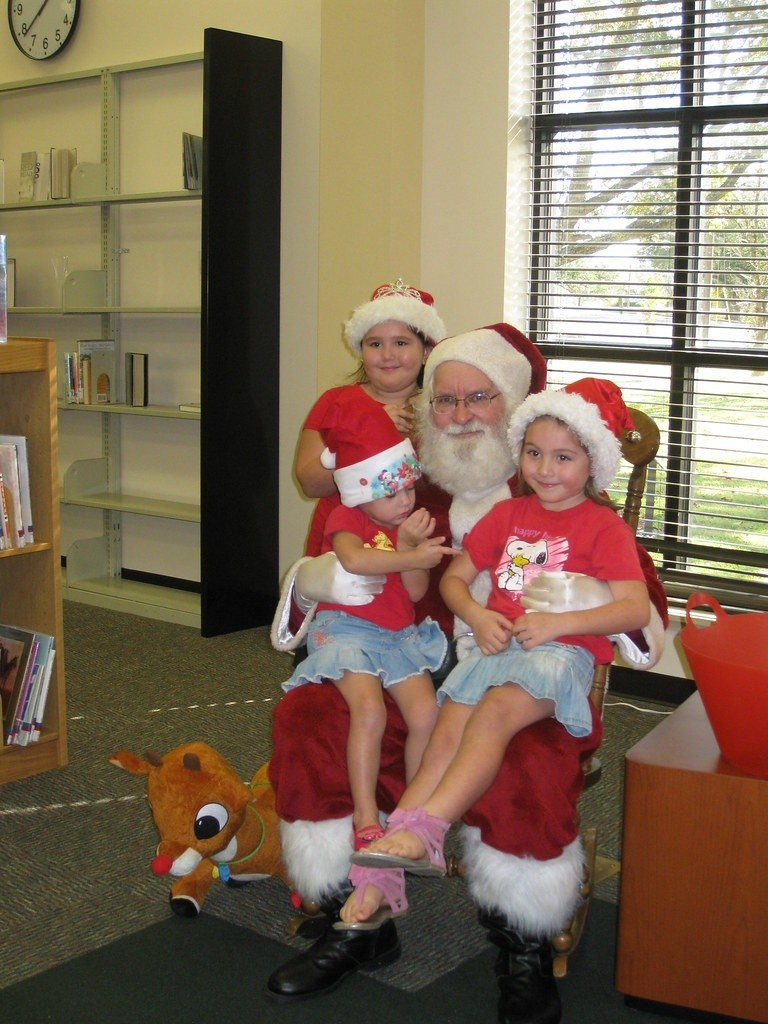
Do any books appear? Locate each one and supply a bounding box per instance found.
[179,402,201,414]
[125,351,149,407]
[0,148,79,205]
[0,623,57,746]
[64,338,116,405]
[0,431,35,552]
[6,256,17,308]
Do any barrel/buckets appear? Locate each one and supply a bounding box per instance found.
[680,592,768,779]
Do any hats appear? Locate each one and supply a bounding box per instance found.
[318,389,424,509]
[507,377,641,493]
[344,284,443,360]
[422,322,549,421]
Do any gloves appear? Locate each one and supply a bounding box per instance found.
[521,571,614,617]
[294,551,391,617]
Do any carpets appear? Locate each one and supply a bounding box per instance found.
[0,897,739,1024]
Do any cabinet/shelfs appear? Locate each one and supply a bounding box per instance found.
[0,329,69,785]
[0,26,282,639]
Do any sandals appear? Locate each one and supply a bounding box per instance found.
[351,809,453,879]
[333,848,412,930]
[351,816,384,851]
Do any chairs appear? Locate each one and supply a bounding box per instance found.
[284,404,661,979]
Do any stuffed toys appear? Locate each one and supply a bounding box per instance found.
[108,741,301,916]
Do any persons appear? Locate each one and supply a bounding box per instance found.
[332,380,651,931]
[291,277,450,548]
[270,323,668,1024]
[279,400,464,848]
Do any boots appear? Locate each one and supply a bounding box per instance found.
[265,877,403,1000]
[479,903,561,1024]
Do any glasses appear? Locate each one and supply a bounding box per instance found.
[428,388,504,417]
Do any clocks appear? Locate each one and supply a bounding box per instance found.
[7,0,81,61]
